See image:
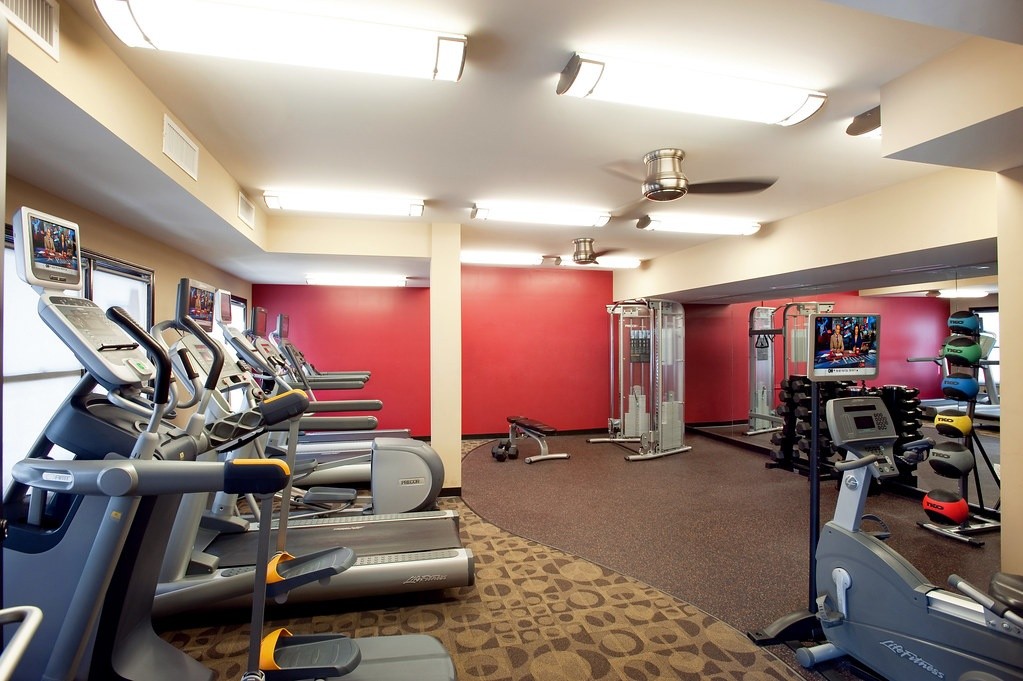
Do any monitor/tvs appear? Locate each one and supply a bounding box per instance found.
[277,314,289,338]
[808,313,881,380]
[215,289,232,324]
[179,278,216,332]
[251,307,268,336]
[13,206,83,291]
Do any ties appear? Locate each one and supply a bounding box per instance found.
[837,335,839,346]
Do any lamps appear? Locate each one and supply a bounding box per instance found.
[928,290,989,298]
[637,212,762,237]
[846,105,883,143]
[535,255,641,269]
[264,190,425,219]
[93,0,470,83]
[304,275,407,287]
[470,200,612,228]
[556,52,828,128]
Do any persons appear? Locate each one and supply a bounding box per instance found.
[190,286,213,321]
[814,317,875,368]
[30,217,76,271]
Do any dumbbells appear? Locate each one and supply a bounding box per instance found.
[765,374,923,498]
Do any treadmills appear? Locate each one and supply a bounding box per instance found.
[0,206,478,680]
[903,331,999,428]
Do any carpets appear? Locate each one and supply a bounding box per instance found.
[110,438,806,680]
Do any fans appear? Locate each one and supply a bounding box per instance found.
[598,149,780,217]
[538,238,625,265]
